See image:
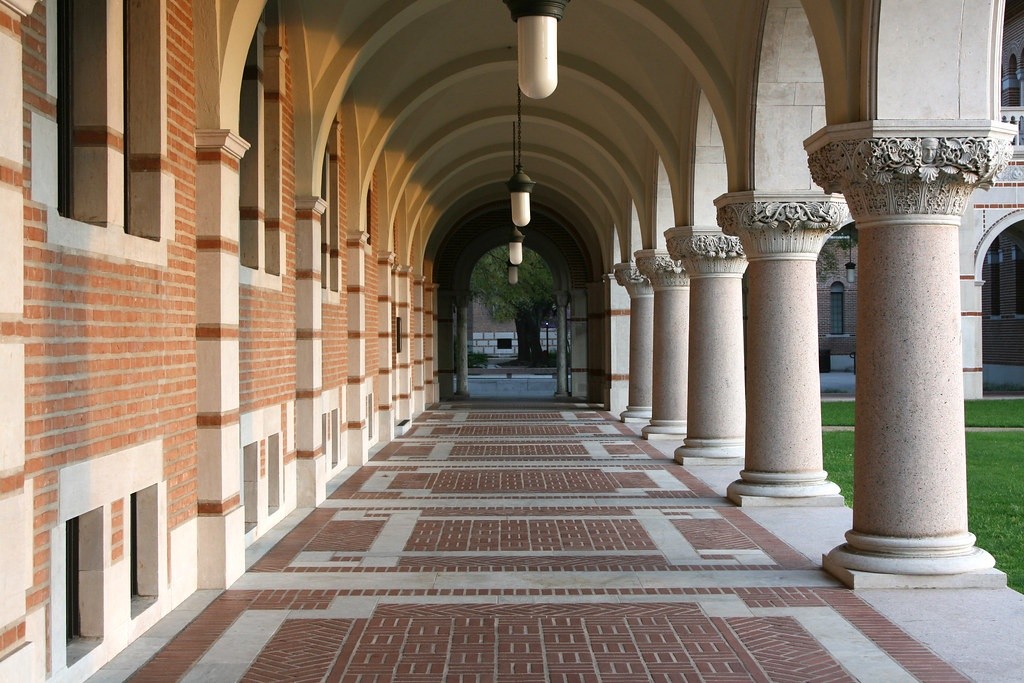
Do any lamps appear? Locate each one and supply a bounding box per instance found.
[503,0,571,100]
[507,258,520,285]
[505,84,536,227]
[845,234,856,283]
[507,121,525,265]
